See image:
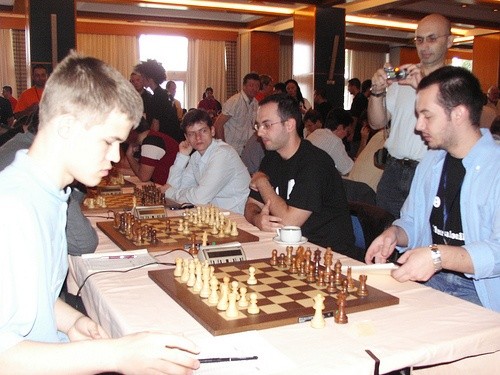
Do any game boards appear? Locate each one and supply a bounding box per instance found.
[148,255,399,336]
[96,214,259,252]
[98,174,137,189]
[79,193,166,214]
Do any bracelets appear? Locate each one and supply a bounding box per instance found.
[430,243,441,274]
[369,85,386,97]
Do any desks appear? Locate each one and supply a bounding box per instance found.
[63,180,500,375]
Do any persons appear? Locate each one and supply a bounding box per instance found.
[122,59,222,183]
[0,63,98,255]
[214,74,372,174]
[0,52,201,375]
[244,93,359,260]
[478,85,499,134]
[364,66,500,313]
[366,14,458,263]
[165,108,254,215]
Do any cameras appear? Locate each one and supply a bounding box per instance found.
[384,68,408,81]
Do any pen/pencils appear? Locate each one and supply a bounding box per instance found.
[199,356,258,363]
[103,254,137,259]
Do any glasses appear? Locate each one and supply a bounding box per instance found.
[412,32,450,44]
[251,120,288,131]
